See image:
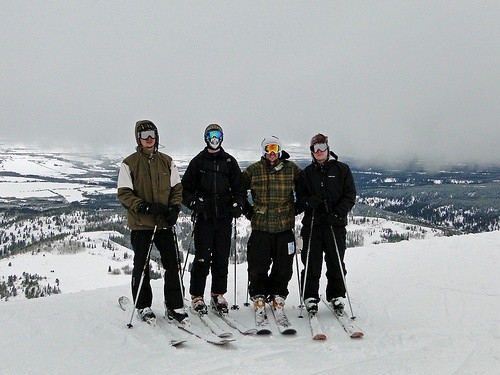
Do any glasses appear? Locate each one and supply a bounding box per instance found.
[139,131,156,140]
[205,130,223,139]
[311,142,329,153]
[265,145,280,153]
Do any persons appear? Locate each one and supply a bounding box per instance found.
[180,125,242,316]
[237,135,307,310]
[116,120,188,324]
[296,134,356,317]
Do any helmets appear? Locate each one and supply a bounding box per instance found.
[261,136,282,158]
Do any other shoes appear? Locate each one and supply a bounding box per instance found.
[138,307,155,323]
[305,297,318,312]
[332,298,344,309]
[253,295,265,308]
[212,295,227,313]
[166,308,188,323]
[274,295,284,309]
[191,295,207,313]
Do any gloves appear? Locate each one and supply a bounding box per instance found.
[230,204,242,218]
[293,200,305,215]
[243,204,255,221]
[190,197,205,211]
[145,204,164,217]
[167,207,179,226]
[326,211,341,224]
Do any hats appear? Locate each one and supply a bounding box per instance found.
[138,123,156,132]
[310,134,328,146]
[205,124,223,134]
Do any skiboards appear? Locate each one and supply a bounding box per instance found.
[300,291,365,340]
[196,309,259,338]
[254,303,297,335]
[117,295,237,347]
